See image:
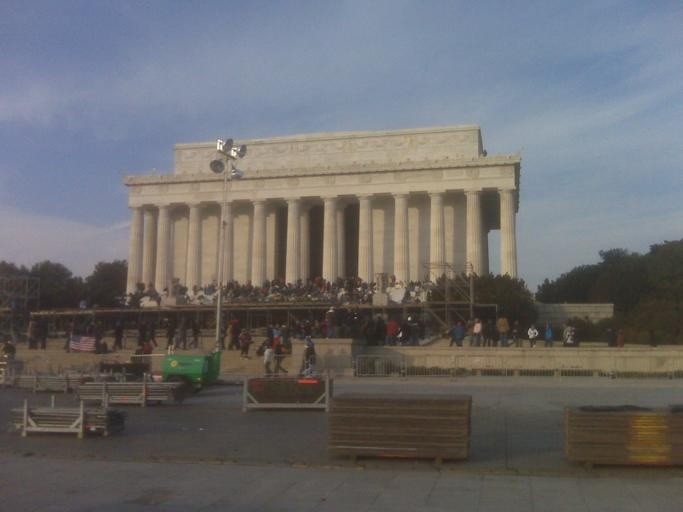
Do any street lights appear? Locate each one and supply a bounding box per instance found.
[208,137,246,353]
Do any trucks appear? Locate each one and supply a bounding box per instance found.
[80,345,219,394]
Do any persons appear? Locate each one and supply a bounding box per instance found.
[648,330,657,347]
[1,275,577,381]
[608,329,618,347]
[617,328,624,347]
